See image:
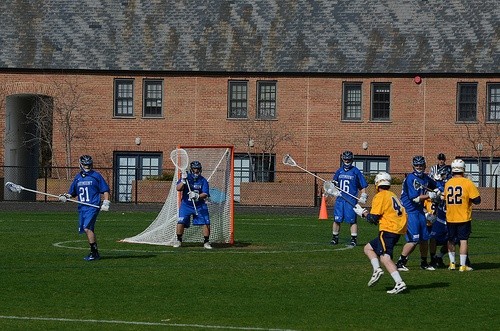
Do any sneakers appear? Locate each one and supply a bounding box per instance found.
[396,255,480,272]
[347,236,358,248]
[203,241,212,250]
[386,281,407,294]
[174,241,182,248]
[330,234,339,245]
[368,267,383,286]
[84,252,100,261]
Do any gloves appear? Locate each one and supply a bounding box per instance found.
[187,191,199,202]
[353,204,366,218]
[100,200,111,212]
[359,192,368,203]
[180,170,188,184]
[58,193,72,204]
[429,188,440,199]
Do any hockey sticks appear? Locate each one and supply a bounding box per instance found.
[170,149,198,215]
[416,182,444,196]
[323,181,378,226]
[4,182,101,209]
[282,154,360,201]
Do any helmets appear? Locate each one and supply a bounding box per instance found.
[190,160,202,178]
[437,153,445,159]
[451,158,465,173]
[79,155,93,175]
[374,172,391,186]
[412,155,426,178]
[342,150,354,171]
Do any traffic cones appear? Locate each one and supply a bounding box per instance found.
[318,196,330,220]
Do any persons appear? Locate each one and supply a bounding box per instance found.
[327,150,369,247]
[395,156,444,271]
[173,160,213,250]
[58,155,111,261]
[429,153,473,268]
[353,171,408,295]
[443,159,481,272]
